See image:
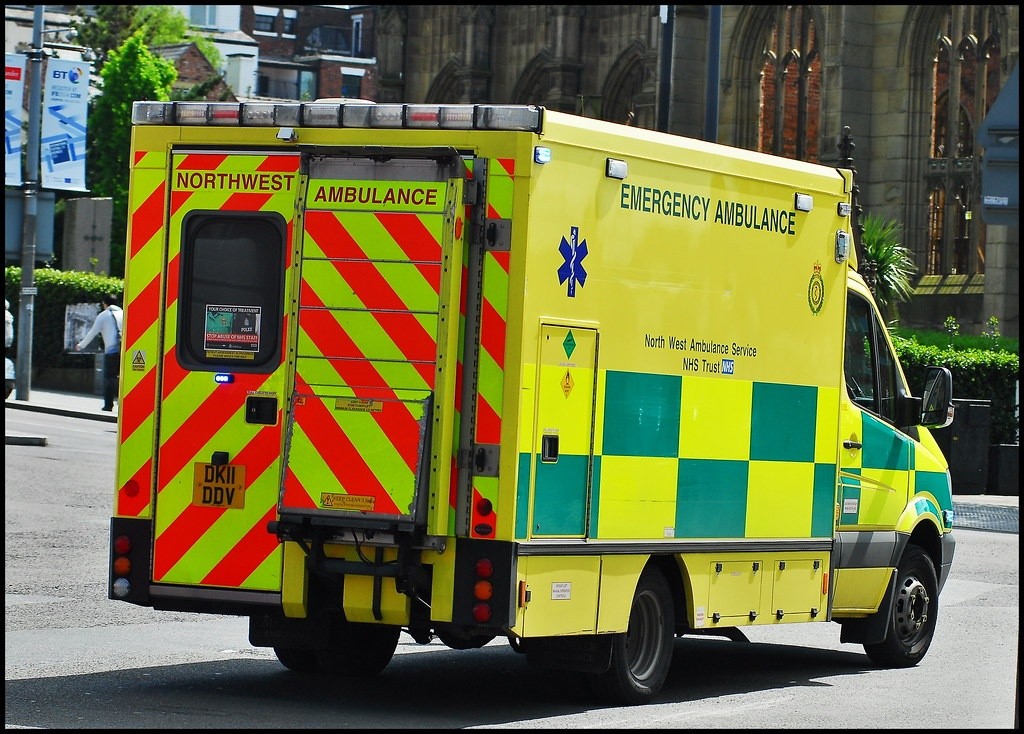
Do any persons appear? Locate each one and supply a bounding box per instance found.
[5,299,14,354]
[75,294,123,412]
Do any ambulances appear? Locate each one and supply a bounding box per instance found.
[108,97,955,699]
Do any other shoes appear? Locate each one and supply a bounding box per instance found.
[102,406,112,411]
